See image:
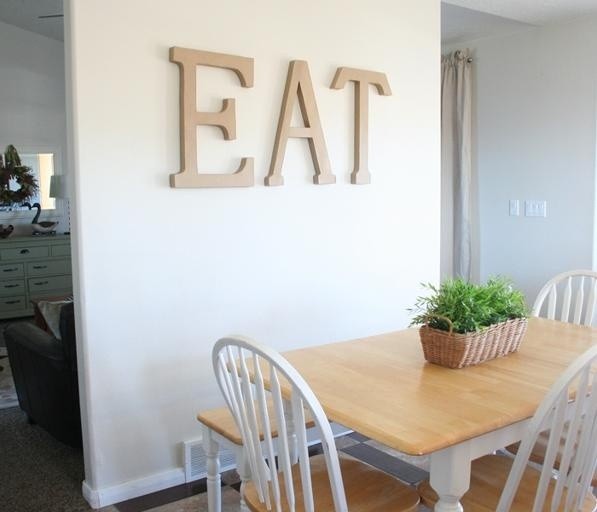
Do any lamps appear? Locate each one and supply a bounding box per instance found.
[50,175,71,235]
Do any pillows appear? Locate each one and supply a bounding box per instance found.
[30,295,73,335]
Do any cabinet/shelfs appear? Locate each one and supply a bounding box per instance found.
[0,235,71,320]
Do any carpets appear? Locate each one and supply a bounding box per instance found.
[0,346,19,410]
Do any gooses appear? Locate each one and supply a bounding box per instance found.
[31,203,59,235]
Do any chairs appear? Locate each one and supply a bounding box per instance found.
[214,337,421,512]
[3,303,80,448]
[506,270,597,486]
[417,345,597,512]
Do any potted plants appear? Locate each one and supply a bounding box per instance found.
[406,274,531,369]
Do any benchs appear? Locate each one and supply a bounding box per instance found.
[197,390,333,511]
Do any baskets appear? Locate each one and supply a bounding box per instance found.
[419,308,529,367]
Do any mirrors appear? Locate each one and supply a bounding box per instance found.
[0,145,62,220]
